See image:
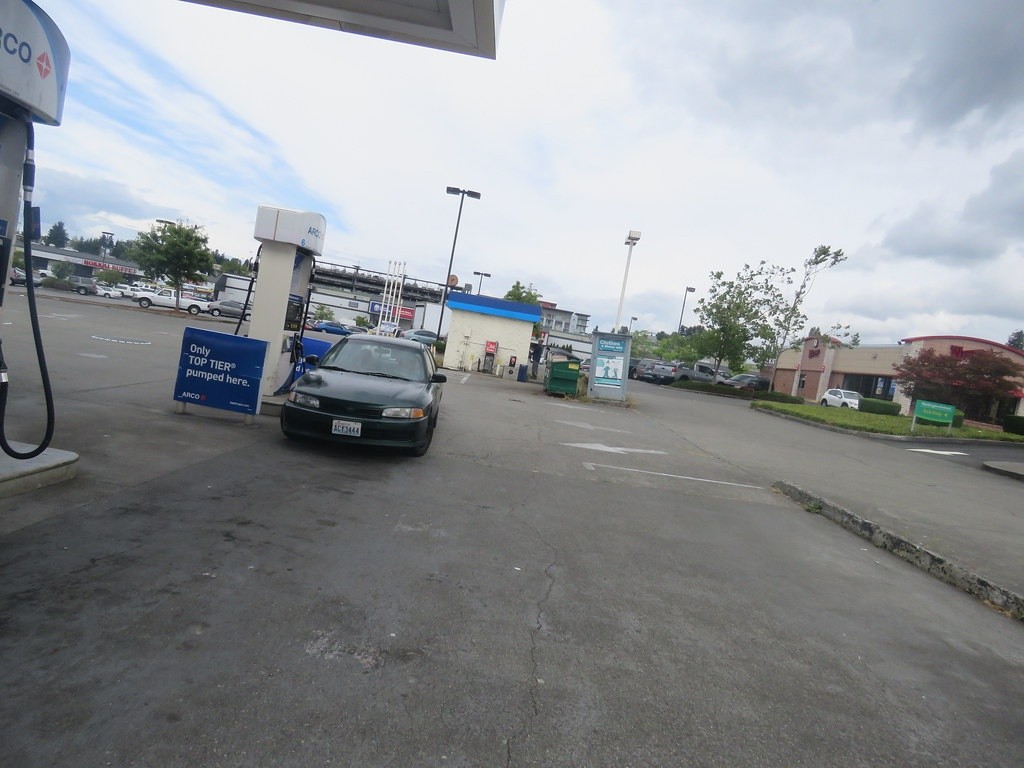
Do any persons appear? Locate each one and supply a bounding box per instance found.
[530,338,544,379]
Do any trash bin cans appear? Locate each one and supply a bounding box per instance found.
[517,364,528,382]
[544,346,583,397]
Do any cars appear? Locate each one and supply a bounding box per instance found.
[579,358,591,373]
[399,328,445,346]
[9,266,252,322]
[302,317,404,338]
[628,356,775,394]
[820,387,864,411]
[278,333,447,458]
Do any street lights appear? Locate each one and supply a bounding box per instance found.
[672,287,696,335]
[628,317,637,333]
[613,230,641,334]
[433,186,481,342]
[474,271,492,295]
